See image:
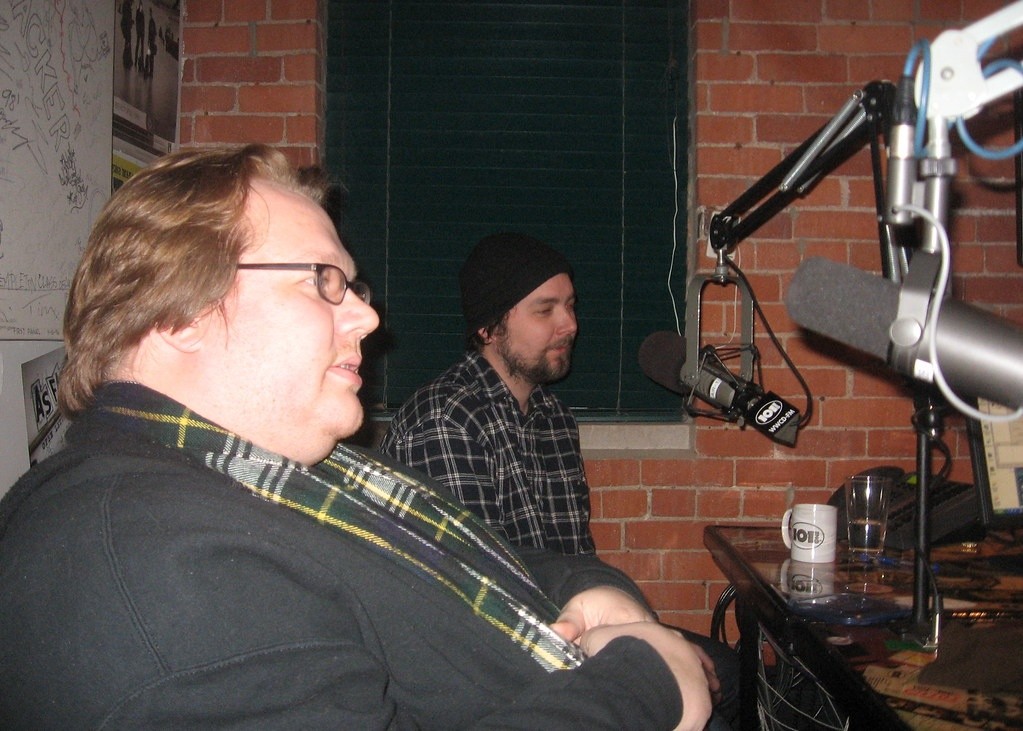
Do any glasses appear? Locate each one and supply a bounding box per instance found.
[233,262,371,309]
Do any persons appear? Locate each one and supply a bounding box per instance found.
[377,233,826,731]
[0,141,721,731]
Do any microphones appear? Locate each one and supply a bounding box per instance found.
[638,331,801,446]
[785,255,1023,413]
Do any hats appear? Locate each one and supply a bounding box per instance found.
[459,231,570,336]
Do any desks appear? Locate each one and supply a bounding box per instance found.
[702,524,1023,731]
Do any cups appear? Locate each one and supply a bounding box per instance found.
[846,475,891,556]
[781,558,834,598]
[782,503,838,563]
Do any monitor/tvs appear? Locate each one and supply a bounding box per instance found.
[963,393,1023,530]
